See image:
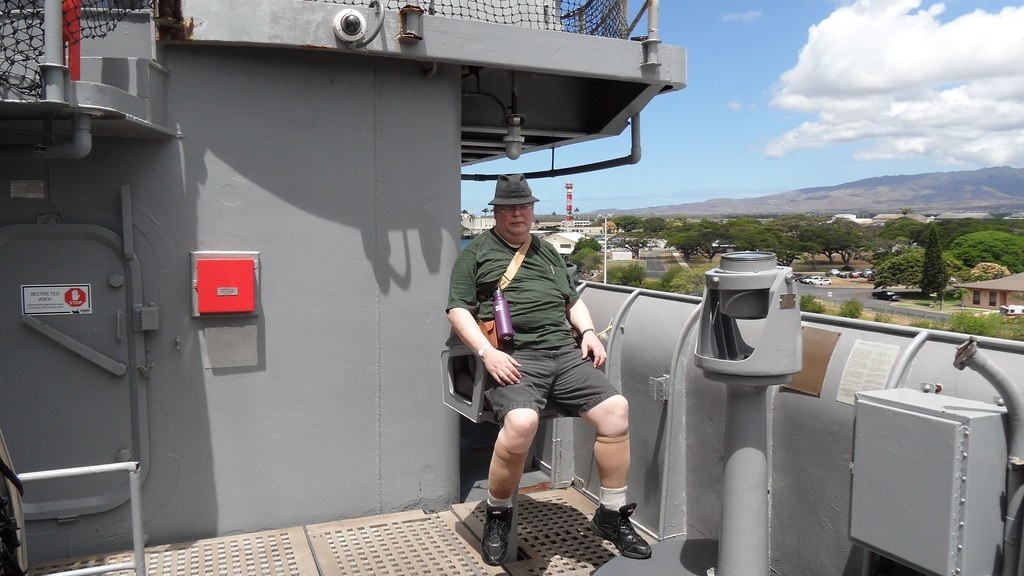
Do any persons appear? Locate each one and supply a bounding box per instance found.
[446,174,652,565]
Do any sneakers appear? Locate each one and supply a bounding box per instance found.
[589,503,652,559]
[480,499,513,566]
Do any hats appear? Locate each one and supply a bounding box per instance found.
[488,173,540,205]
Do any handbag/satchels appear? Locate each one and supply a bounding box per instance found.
[445,320,503,350]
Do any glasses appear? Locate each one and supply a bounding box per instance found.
[495,205,533,215]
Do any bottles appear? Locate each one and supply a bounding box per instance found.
[493,285,514,340]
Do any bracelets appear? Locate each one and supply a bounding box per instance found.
[582,328,598,337]
[478,344,492,358]
